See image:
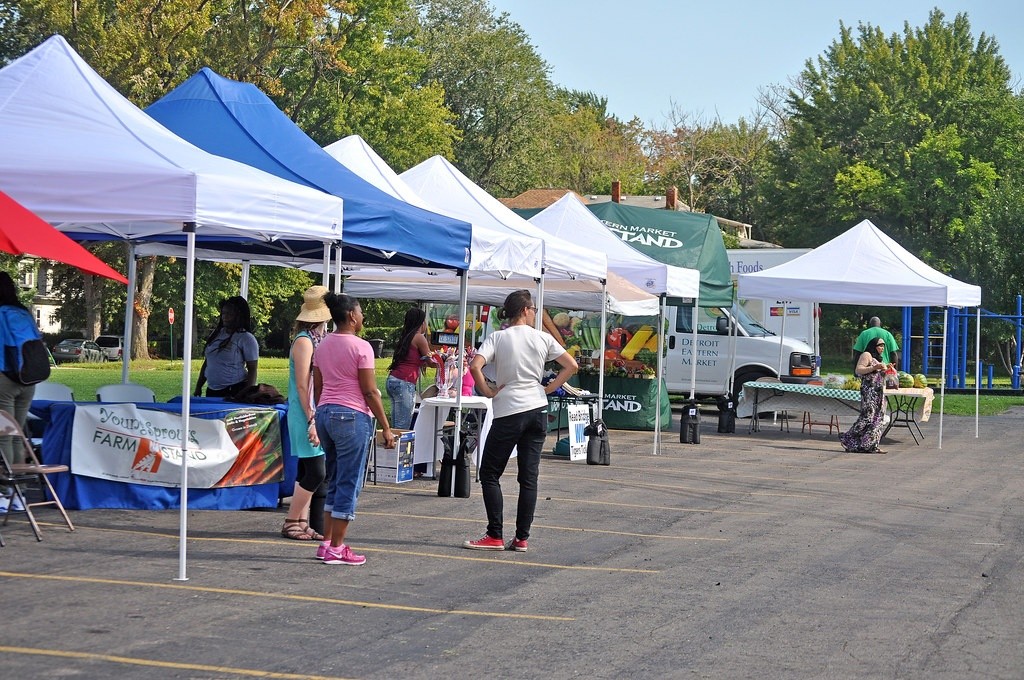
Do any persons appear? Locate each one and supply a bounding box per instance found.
[279,286,332,541]
[387,308,431,430]
[462,290,578,552]
[841,337,892,454]
[194,296,259,397]
[311,291,395,565]
[0,272,41,515]
[853,317,899,378]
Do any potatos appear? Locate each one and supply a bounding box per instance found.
[552,312,581,331]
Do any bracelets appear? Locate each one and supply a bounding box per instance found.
[308,415,315,425]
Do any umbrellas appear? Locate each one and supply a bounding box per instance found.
[0,193,128,284]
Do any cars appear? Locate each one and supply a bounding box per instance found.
[52,338,110,366]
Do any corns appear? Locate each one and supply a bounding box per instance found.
[621,324,664,360]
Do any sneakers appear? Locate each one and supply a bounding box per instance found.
[504,536,528,552]
[322,544,366,566]
[462,533,505,551]
[314,540,331,560]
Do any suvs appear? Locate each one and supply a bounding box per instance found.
[95,335,124,361]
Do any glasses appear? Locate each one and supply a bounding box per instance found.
[522,305,539,313]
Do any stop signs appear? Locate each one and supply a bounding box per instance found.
[169,308,174,324]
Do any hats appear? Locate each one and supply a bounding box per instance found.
[877,338,886,346]
[296,285,337,323]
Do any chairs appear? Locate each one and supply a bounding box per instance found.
[410,385,456,437]
[0,382,156,546]
[756,377,840,435]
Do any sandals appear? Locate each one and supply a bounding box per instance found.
[298,519,325,541]
[281,519,313,541]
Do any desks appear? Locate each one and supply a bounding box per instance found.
[545,375,673,440]
[29,396,299,510]
[736,381,935,445]
[413,395,518,482]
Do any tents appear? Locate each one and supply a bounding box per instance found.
[64,67,472,497]
[0,34,342,579]
[737,219,981,448]
[137,135,701,455]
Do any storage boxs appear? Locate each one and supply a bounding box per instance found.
[371,428,416,484]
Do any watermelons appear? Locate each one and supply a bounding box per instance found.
[898,372,927,387]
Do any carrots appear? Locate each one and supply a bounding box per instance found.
[213,433,283,485]
[542,309,566,348]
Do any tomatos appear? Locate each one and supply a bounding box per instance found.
[442,313,460,332]
[605,327,632,359]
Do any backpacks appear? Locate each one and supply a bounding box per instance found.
[0,305,50,386]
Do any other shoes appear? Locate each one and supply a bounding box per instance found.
[0,491,28,515]
[875,449,889,454]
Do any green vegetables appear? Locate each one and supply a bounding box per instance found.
[563,314,603,350]
[633,344,667,366]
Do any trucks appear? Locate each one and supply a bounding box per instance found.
[724,248,821,379]
[478,301,824,419]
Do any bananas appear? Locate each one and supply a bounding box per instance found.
[841,377,862,391]
